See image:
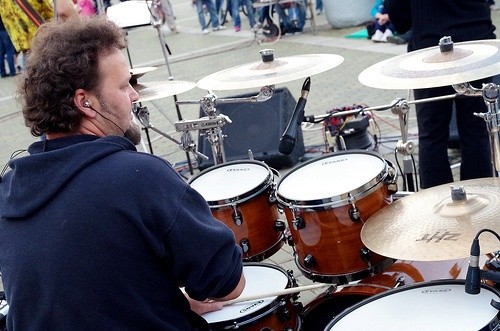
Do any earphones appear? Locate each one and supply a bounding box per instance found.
[83,100,90,109]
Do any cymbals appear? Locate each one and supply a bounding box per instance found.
[359,176,500,262]
[127,67,196,104]
[195,53,344,91]
[358,39,500,90]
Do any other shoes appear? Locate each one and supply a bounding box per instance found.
[171,29,179,33]
[380,31,393,41]
[387,35,405,44]
[235,26,241,32]
[254,23,263,28]
[372,31,384,40]
[3,74,10,77]
[10,72,22,76]
[203,29,209,33]
[213,25,226,31]
[284,29,302,35]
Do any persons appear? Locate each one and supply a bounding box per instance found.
[194,0,323,37]
[0,0,130,77]
[151,0,180,33]
[0,17,246,331]
[371,0,497,189]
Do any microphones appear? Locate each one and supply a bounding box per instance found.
[464,239,480,294]
[279,80,311,155]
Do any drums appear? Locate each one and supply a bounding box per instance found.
[183,147,500,331]
